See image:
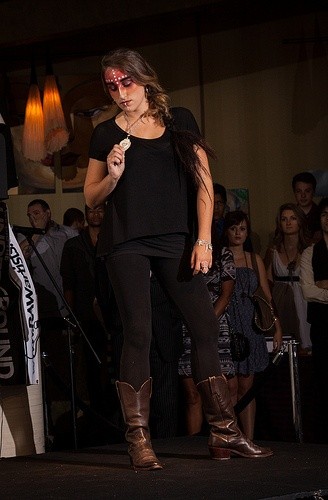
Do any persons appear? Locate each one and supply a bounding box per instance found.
[0,199,124,419]
[148,172,328,442]
[84,48,275,471]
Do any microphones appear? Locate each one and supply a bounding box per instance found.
[11,225,47,236]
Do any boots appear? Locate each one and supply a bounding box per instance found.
[196,374,274,461]
[114,377,163,471]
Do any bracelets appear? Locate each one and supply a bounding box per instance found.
[195,238,213,251]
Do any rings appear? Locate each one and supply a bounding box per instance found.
[199,262,209,274]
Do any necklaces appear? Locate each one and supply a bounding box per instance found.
[119,110,145,151]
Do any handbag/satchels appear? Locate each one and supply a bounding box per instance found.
[252,286,277,336]
